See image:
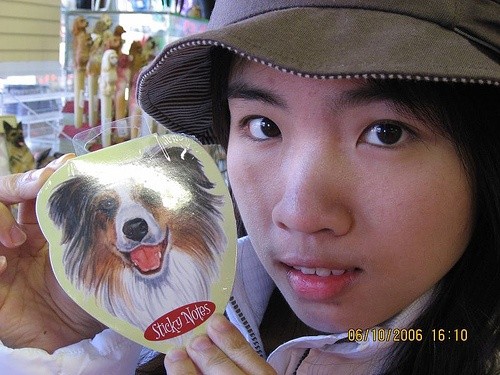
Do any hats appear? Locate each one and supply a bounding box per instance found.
[135,0,499,146]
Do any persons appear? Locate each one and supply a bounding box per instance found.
[0,0,500,375]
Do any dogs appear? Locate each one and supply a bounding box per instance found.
[47,145,228,348]
[72,16,94,72]
[3,120,37,209]
